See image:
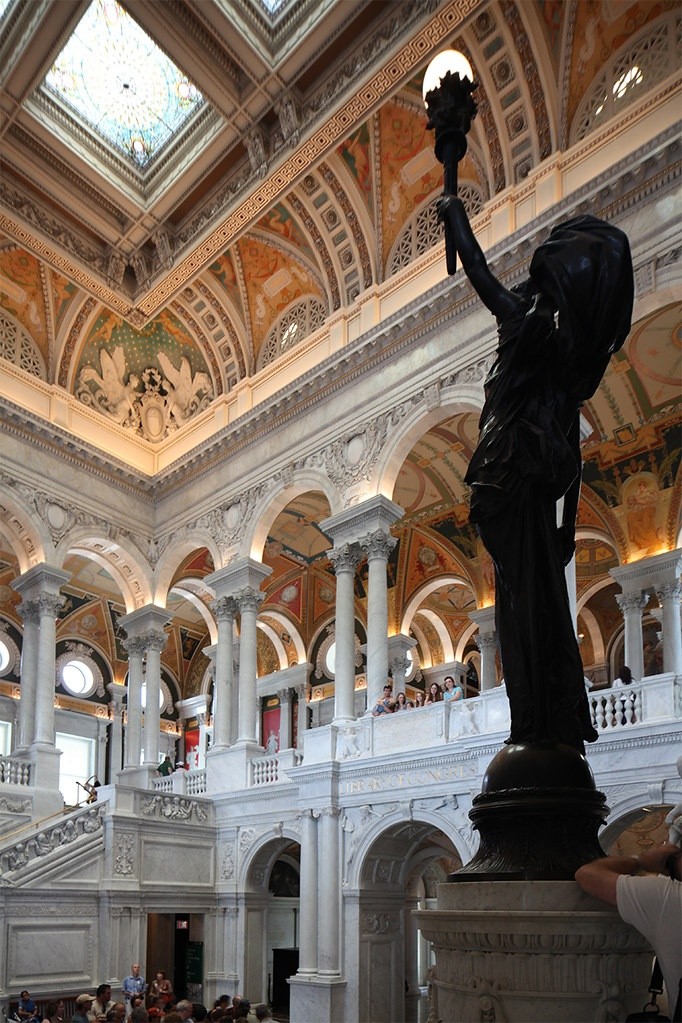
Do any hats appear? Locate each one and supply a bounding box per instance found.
[76,994,97,1005]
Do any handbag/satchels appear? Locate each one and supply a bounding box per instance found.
[624,1002,672,1023]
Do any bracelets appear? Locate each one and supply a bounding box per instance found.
[628,854,641,868]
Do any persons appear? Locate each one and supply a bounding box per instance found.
[644,640,661,677]
[265,729,280,755]
[18,962,282,1023]
[372,676,463,717]
[612,666,636,726]
[574,843,682,1023]
[156,756,188,778]
[433,196,635,744]
[161,378,211,428]
[91,374,144,425]
